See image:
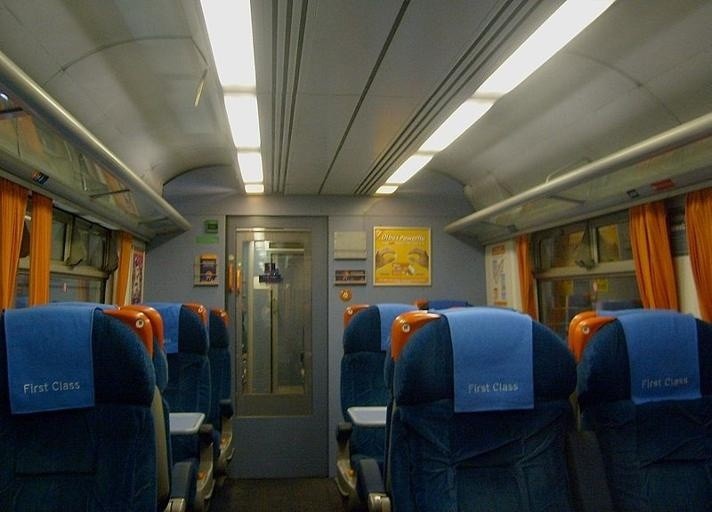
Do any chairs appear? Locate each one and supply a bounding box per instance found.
[2,298,243,511]
[331,295,712,512]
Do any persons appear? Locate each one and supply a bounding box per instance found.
[375,247,428,273]
